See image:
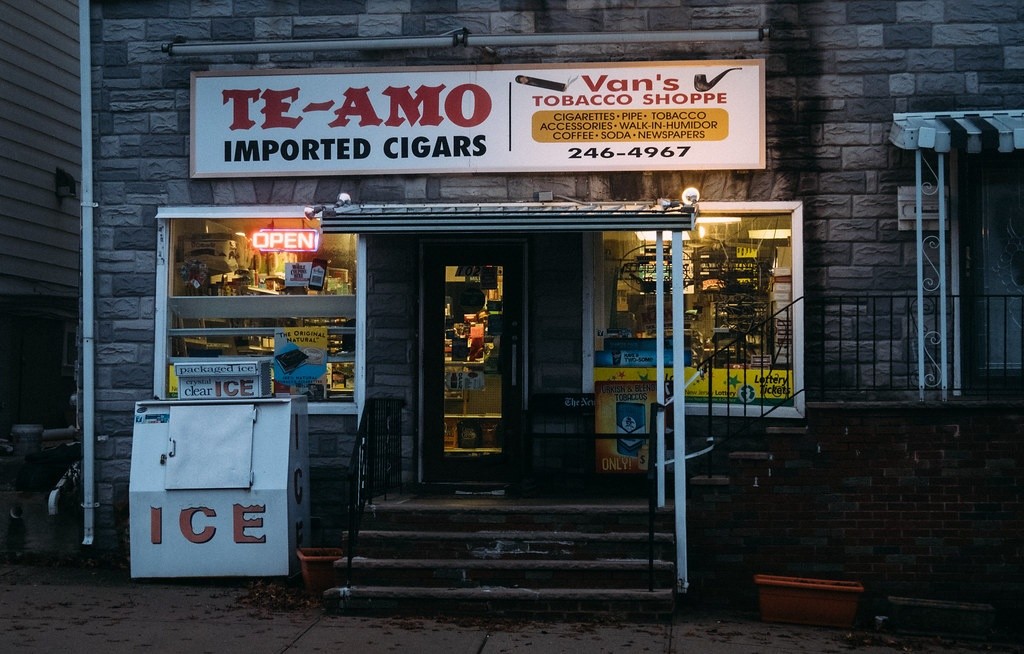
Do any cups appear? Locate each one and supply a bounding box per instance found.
[308,258,327,290]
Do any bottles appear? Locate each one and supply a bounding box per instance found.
[451,373,458,388]
[637,444,650,478]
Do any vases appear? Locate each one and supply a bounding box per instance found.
[887,595,995,642]
[753,574,863,629]
[295,547,342,590]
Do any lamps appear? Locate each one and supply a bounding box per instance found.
[161,28,773,56]
